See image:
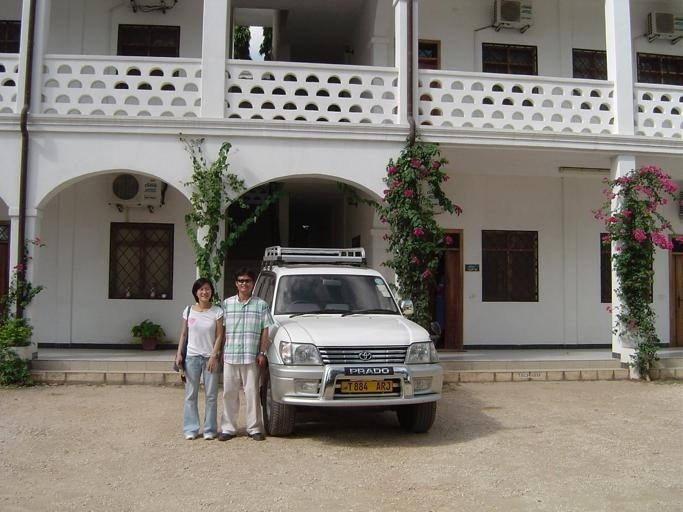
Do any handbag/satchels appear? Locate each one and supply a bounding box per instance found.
[173,342,187,372]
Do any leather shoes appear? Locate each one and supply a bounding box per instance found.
[218,433,236,441]
[248,433,264,441]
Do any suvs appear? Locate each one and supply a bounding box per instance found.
[251,245,443,435]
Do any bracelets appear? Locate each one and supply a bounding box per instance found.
[259,351,268,357]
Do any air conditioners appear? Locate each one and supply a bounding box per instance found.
[491,1,533,33]
[106,171,165,212]
[649,12,682,44]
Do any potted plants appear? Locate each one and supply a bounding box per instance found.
[132,319,164,348]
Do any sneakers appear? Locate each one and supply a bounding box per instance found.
[185,434,195,440]
[204,433,216,440]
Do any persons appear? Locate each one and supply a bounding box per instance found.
[174,278,222,441]
[217,269,274,445]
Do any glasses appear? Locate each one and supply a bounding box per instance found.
[238,279,252,283]
